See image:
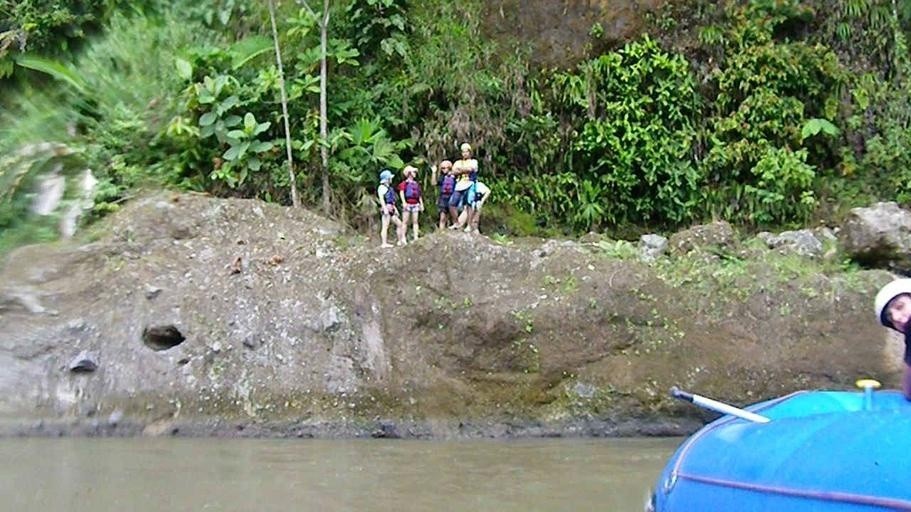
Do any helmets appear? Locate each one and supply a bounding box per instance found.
[439,159,453,170]
[874,277,911,331]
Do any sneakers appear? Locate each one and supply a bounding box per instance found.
[375,221,485,250]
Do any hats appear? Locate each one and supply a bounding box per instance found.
[379,169,396,185]
[403,165,419,178]
[460,142,473,152]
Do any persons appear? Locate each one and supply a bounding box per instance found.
[431,160,458,233]
[872,276,911,403]
[448,180,493,234]
[447,140,481,233]
[376,169,407,249]
[397,164,426,245]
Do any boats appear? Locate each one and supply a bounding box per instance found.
[644,385,910,512]
